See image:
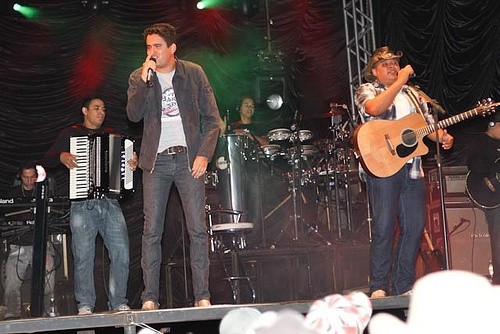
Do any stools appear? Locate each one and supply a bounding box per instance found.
[206,208,256,305]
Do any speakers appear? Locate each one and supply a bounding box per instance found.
[429,201,494,281]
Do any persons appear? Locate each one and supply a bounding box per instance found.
[42,97,140,315]
[219,270,500,334]
[354,45,455,300]
[3,164,62,319]
[467,117,500,285]
[127,23,226,310]
[215,92,271,153]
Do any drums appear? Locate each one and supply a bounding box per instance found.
[285,146,319,158]
[251,145,281,159]
[281,170,318,191]
[293,130,314,140]
[208,134,262,173]
[334,141,360,175]
[267,128,291,141]
[310,138,335,176]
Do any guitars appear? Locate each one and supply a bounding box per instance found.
[465,158,500,209]
[354,98,500,179]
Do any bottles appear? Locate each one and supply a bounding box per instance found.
[488,260,494,278]
[50,298,57,317]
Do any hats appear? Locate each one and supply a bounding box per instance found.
[305,291,373,334]
[365,46,403,82]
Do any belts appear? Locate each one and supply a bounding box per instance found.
[165,146,187,155]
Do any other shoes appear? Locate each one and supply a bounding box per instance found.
[371,290,386,298]
[194,299,211,307]
[4,311,20,318]
[114,304,130,311]
[141,301,159,310]
[399,290,412,296]
[78,306,92,315]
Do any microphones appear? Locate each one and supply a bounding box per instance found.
[290,110,298,131]
[395,71,416,78]
[147,58,156,82]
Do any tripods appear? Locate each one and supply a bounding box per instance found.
[269,122,332,251]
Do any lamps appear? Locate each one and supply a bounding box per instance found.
[253,49,288,111]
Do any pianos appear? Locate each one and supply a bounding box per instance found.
[0,195,70,318]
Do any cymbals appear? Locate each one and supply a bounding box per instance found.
[317,110,344,117]
